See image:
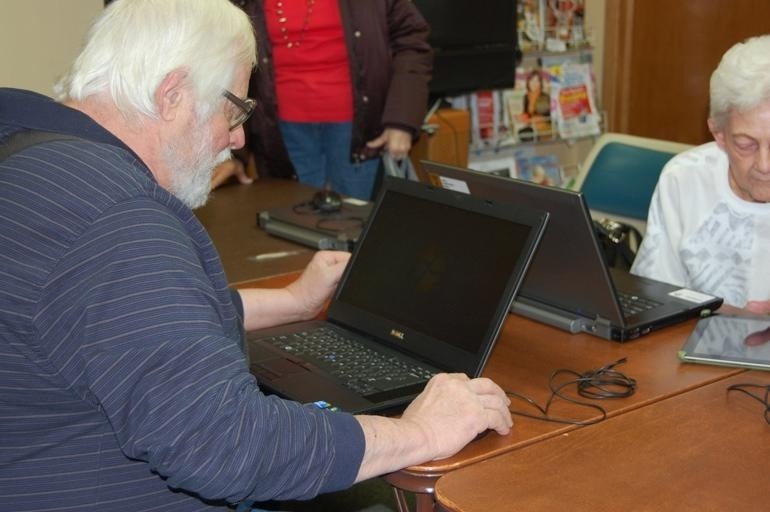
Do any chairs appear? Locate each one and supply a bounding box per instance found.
[571,130,698,241]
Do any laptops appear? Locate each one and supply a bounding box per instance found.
[421,160,724,344]
[246,175,551,417]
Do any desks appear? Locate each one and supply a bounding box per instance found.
[196,175,769,512]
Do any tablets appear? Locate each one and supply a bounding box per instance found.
[678,313,770,370]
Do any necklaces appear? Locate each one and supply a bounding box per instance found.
[274,0,316,50]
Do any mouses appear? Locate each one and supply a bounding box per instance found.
[313,190,343,211]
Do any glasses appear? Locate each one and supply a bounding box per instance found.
[220,86,258,133]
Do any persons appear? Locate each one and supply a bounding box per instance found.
[626,31,769,352]
[517,70,554,137]
[1,2,516,511]
[238,1,436,202]
